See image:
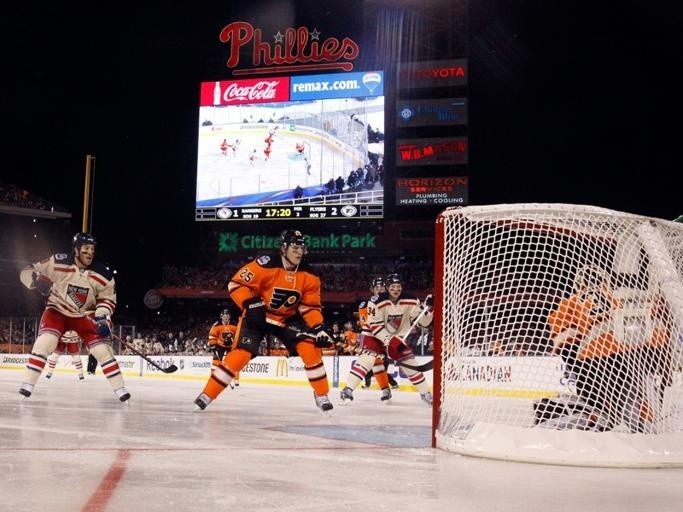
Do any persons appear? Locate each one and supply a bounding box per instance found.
[192,229,336,412]
[1,179,56,211]
[2,320,38,355]
[451,339,523,354]
[315,258,434,291]
[124,327,210,355]
[361,357,399,390]
[208,309,241,387]
[42,330,85,382]
[257,337,288,356]
[322,322,361,354]
[534,262,674,435]
[218,121,383,202]
[16,231,133,403]
[342,271,436,404]
[356,275,393,401]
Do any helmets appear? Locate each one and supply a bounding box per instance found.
[372,277,386,287]
[386,273,401,285]
[71,233,96,248]
[280,229,308,255]
[575,265,606,289]
[220,309,231,316]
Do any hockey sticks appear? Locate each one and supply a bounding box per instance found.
[385,295,434,388]
[213,349,235,391]
[31,271,178,373]
[265,318,434,371]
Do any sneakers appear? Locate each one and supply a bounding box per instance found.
[316,396,333,410]
[420,391,433,404]
[196,394,211,410]
[342,387,354,401]
[19,383,34,397]
[115,387,130,402]
[381,389,392,400]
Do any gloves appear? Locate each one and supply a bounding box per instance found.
[312,326,338,347]
[243,297,268,330]
[94,315,113,337]
[34,275,53,296]
